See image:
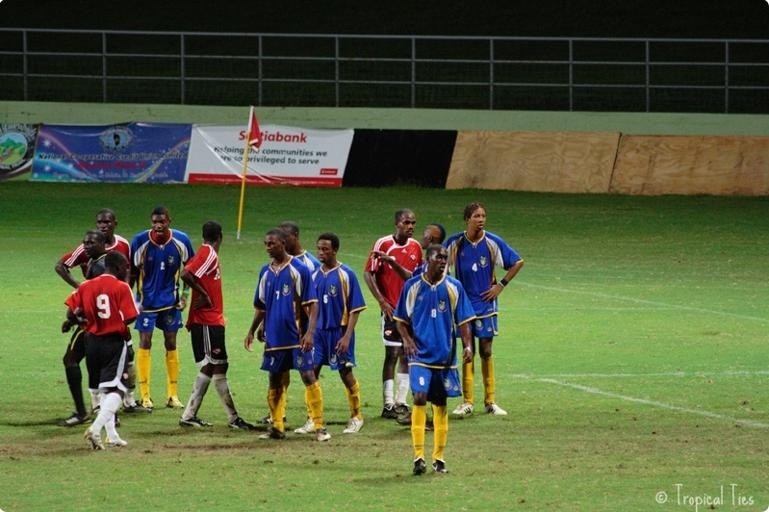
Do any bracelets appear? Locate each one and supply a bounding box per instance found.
[499,279,509,287]
[466,346,473,350]
[180,287,189,300]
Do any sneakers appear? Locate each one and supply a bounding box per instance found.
[431,458,448,473]
[83,428,106,450]
[139,396,154,409]
[104,436,128,447]
[259,402,434,442]
[57,405,152,427]
[228,417,264,432]
[451,402,474,419]
[484,403,508,416]
[166,396,184,409]
[413,457,426,475]
[178,415,214,429]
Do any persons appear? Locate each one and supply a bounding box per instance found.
[63,250,140,450]
[364,209,424,420]
[127,206,194,409]
[442,203,525,416]
[55,207,152,414]
[56,230,122,429]
[255,222,321,423]
[245,228,331,442]
[178,220,255,432]
[112,133,125,151]
[370,223,446,431]
[294,234,365,434]
[392,241,477,475]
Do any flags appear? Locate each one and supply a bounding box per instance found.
[246,106,264,154]
